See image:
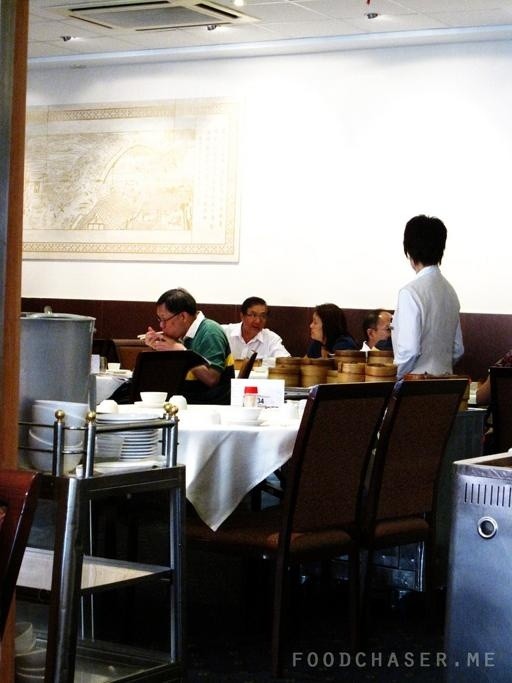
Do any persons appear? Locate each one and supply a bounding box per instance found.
[143,287,236,386]
[218,296,293,368]
[389,214,465,381]
[476,349,512,403]
[359,309,395,353]
[304,302,359,361]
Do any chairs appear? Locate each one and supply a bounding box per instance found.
[490,365,512,451]
[169,380,394,682]
[225,348,259,406]
[120,350,201,400]
[361,377,468,682]
[0,466,45,682]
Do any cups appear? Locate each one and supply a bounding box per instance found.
[101,400,118,412]
[237,406,261,420]
[169,394,188,409]
[107,362,120,369]
[467,381,480,404]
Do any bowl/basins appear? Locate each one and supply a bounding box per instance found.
[140,390,168,405]
[29,397,88,476]
[12,618,50,680]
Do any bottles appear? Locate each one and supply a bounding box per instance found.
[243,385,258,408]
[100,356,107,372]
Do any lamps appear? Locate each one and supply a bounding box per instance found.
[205,24,218,31]
[60,35,76,44]
[366,10,381,22]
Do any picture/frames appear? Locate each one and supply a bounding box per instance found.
[21,98,251,262]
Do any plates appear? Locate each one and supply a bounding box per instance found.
[94,461,155,473]
[94,406,161,462]
[107,369,129,373]
[233,419,264,425]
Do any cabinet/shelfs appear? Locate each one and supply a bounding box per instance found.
[11,402,190,682]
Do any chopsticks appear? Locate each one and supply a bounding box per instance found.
[137,331,165,339]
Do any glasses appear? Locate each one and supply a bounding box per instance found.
[246,313,267,322]
[156,313,178,323]
